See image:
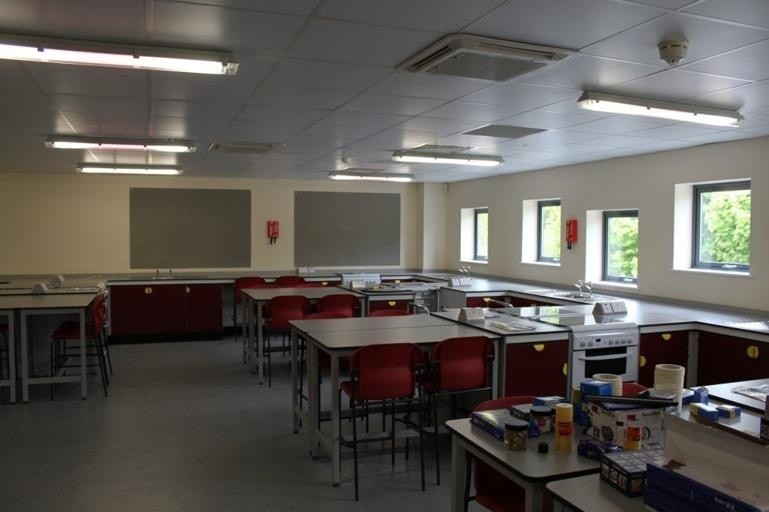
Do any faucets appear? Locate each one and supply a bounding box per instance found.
[462,266,471,276]
[455,265,464,274]
[168,269,172,277]
[156,270,159,277]
[584,281,593,296]
[573,280,583,293]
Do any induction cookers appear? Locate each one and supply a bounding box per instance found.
[536,315,632,327]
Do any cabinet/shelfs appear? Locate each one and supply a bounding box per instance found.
[104,282,189,342]
[189,282,222,339]
[636,326,689,388]
[510,297,550,308]
[696,325,769,387]
[468,297,504,308]
[503,334,570,402]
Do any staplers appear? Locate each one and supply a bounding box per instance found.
[577,444,605,462]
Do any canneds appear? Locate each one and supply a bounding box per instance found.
[503,419,528,451]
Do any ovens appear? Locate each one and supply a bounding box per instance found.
[566,326,640,397]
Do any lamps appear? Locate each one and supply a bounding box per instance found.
[75,163,185,178]
[328,168,420,186]
[390,149,504,168]
[0,34,248,76]
[576,87,744,129]
[43,137,195,153]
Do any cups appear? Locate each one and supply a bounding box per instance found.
[503,419,529,451]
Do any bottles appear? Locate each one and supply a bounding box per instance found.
[555,403,574,456]
[623,425,641,452]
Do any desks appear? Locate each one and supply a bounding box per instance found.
[0,290,98,405]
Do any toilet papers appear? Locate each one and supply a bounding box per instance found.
[593,374,623,397]
[653,363,685,416]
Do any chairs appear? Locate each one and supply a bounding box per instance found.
[469,396,556,511]
[50,291,113,400]
[624,383,646,396]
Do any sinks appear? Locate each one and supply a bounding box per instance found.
[554,293,589,299]
[428,274,457,278]
[152,276,175,280]
